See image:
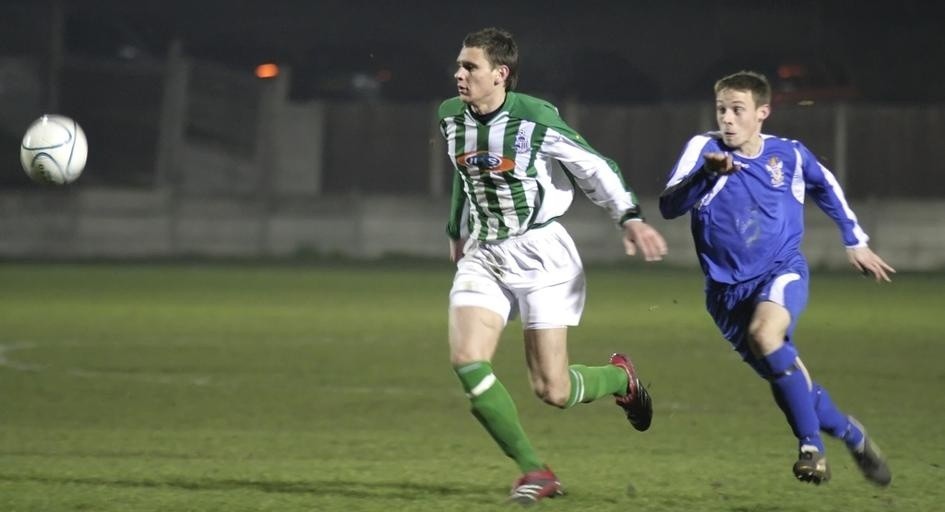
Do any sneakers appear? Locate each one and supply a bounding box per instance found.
[608,352,652,432]
[845,414,893,487]
[506,464,565,505]
[792,444,832,485]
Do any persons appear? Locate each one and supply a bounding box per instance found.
[659,73,896,486]
[438,27,667,507]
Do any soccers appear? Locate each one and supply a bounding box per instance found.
[20,114,89,186]
